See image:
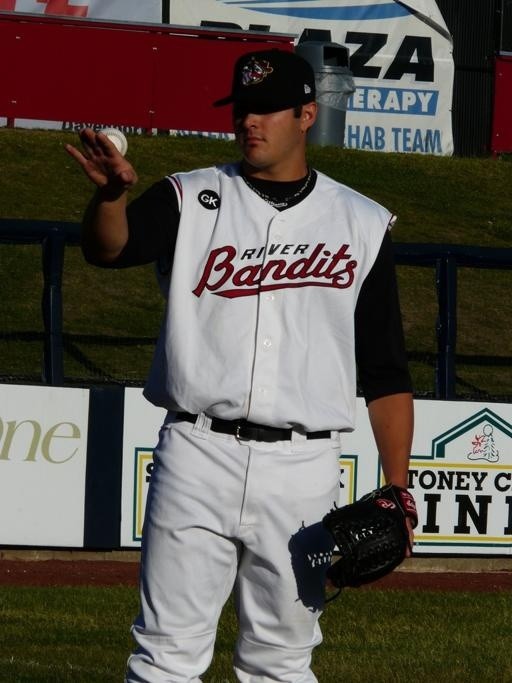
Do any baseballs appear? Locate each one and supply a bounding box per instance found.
[100,128,128,157]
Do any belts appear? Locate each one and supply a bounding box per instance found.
[176,412,331,443]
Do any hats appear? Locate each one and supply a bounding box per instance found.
[213,51,316,115]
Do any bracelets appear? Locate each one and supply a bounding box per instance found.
[394,484,418,530]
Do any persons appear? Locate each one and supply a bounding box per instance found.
[61,43,419,683]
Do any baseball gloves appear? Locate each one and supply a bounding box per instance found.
[323,484,418,589]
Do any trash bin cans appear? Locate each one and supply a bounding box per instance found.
[296,41,350,145]
